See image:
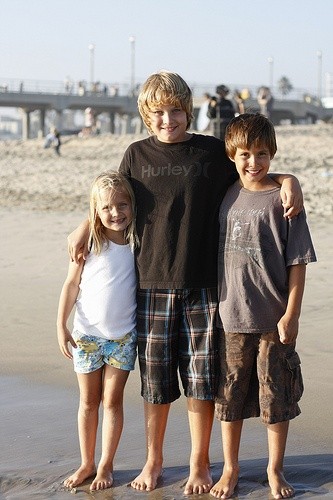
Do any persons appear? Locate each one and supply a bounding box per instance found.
[57,172,141,491]
[67,72,318,499]
[50,127,63,156]
